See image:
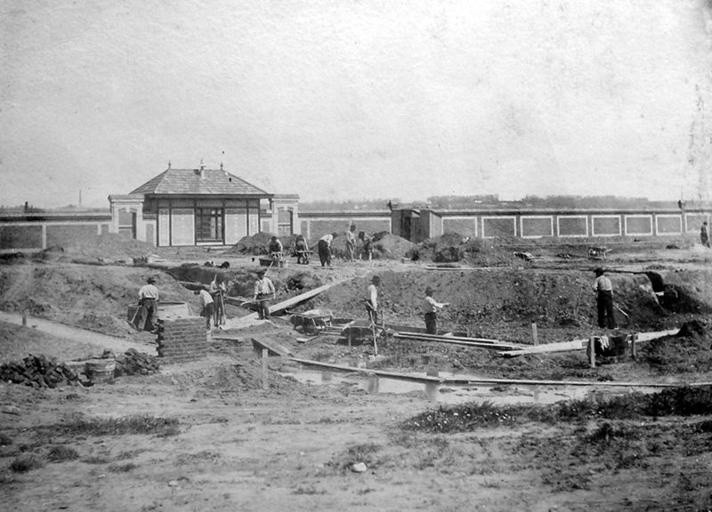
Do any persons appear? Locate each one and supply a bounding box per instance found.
[422,286,451,334]
[199,286,218,330]
[138,276,160,332]
[592,268,615,330]
[346,225,356,261]
[270,236,283,268]
[358,231,373,262]
[209,261,234,326]
[700,221,711,248]
[251,271,275,319]
[296,235,308,265]
[318,232,338,266]
[366,276,380,324]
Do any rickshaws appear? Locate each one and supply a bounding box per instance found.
[285,310,384,345]
[588,246,607,261]
[585,330,638,367]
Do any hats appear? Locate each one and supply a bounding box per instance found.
[256,267,267,274]
[425,286,436,293]
[594,269,606,273]
[370,275,382,281]
[146,277,156,282]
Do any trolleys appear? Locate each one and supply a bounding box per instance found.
[297,249,313,263]
